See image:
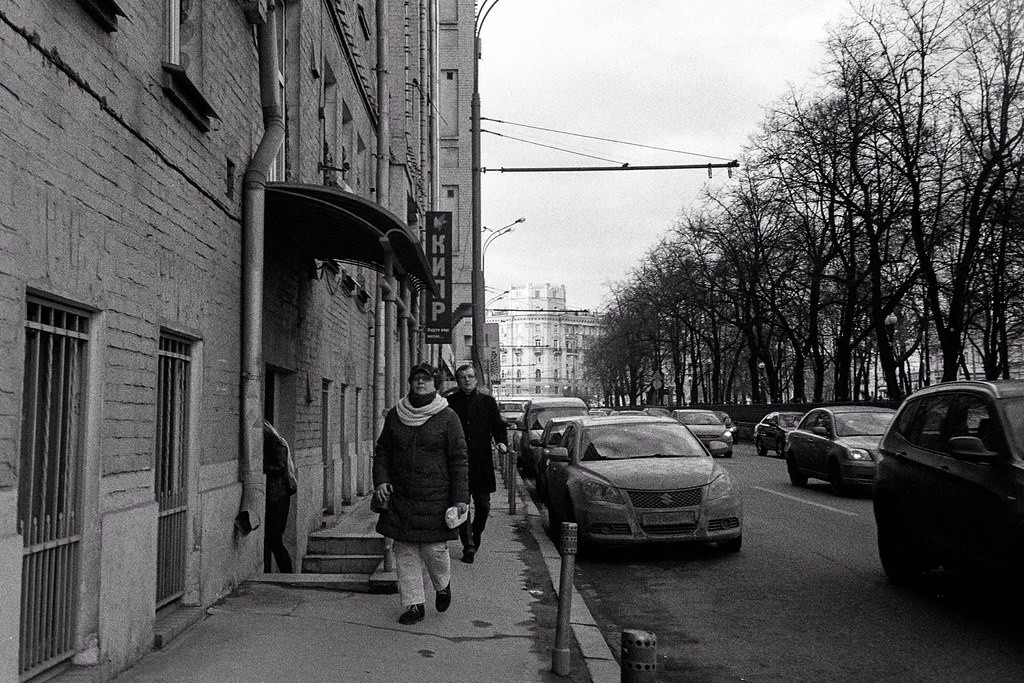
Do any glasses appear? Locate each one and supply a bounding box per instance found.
[413,375,432,381]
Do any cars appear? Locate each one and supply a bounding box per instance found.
[785,406,898,499]
[497,396,672,497]
[753,411,807,457]
[545,415,745,549]
[671,409,733,458]
[714,411,739,445]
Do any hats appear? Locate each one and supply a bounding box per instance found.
[411,362,437,378]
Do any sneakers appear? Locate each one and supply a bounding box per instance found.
[435,581,451,612]
[398,604,425,625]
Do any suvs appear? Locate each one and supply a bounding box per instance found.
[869,381,1024,594]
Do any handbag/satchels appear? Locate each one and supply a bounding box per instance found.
[285,472,297,494]
[371,486,394,514]
[445,505,469,529]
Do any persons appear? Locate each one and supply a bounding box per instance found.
[372,361,470,623]
[446,364,508,564]
[263,420,297,574]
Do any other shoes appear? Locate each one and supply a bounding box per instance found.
[462,552,474,563]
[473,532,481,551]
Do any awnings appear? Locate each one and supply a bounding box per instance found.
[263,181,440,300]
